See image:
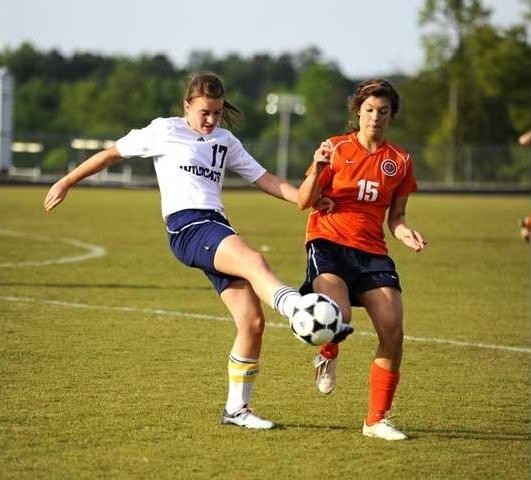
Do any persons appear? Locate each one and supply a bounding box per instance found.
[517,120,531,241]
[297,78,428,443]
[42,71,355,434]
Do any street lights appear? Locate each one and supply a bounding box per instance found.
[264,93,306,180]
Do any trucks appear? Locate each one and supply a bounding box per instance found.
[68,137,133,187]
[6,141,45,184]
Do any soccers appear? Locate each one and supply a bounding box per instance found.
[289,293,342,346]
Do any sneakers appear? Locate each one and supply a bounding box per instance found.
[219,403,274,430]
[328,322,356,345]
[313,349,338,393]
[360,420,408,441]
